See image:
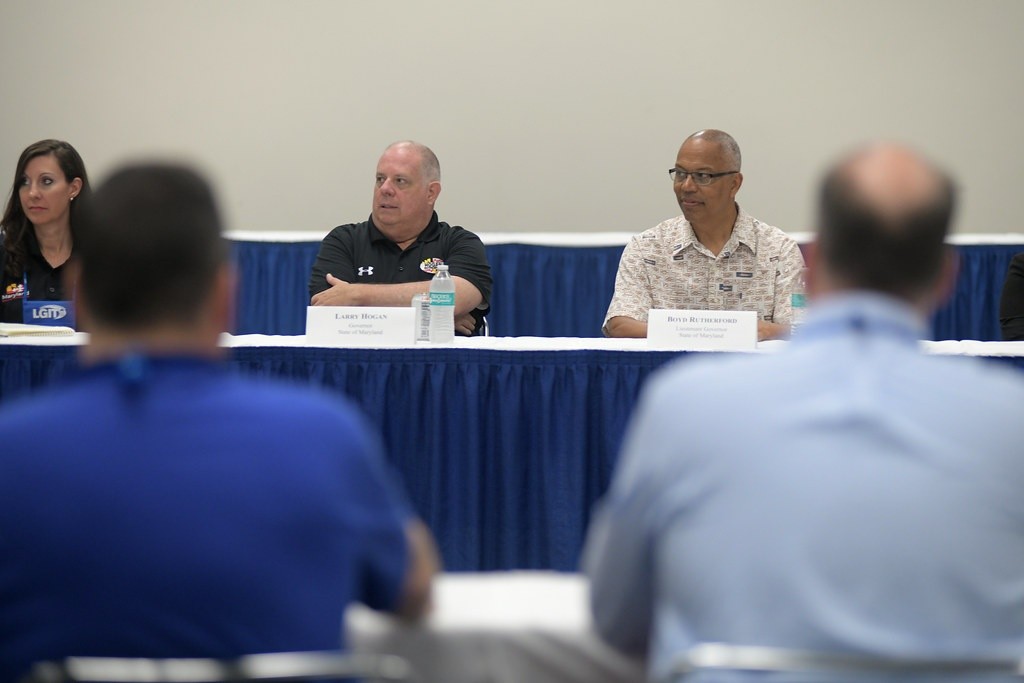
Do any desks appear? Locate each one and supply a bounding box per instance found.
[342,568,642,683]
[0,318,1024,578]
[220,229,1024,340]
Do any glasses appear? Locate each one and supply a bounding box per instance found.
[669,167,738,185]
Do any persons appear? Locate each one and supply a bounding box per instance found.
[601,129,807,342]
[0,139,91,333]
[0,157,434,683]
[581,143,1023,683]
[307,140,493,337]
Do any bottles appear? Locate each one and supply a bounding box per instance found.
[790,269,808,339]
[429,265,455,344]
